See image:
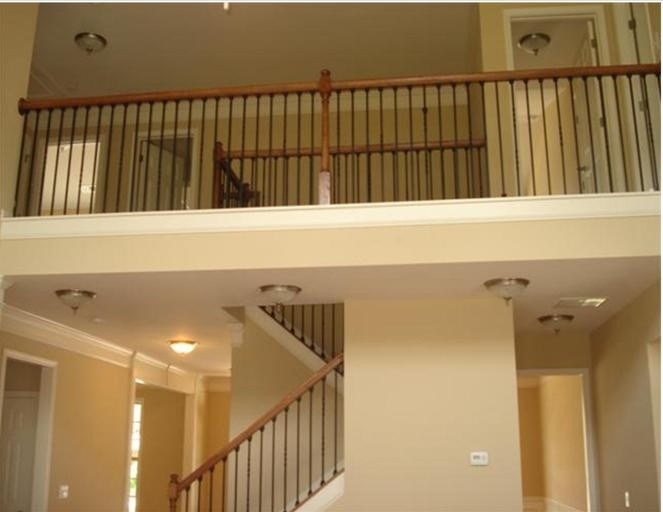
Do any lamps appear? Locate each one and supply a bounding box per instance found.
[519,31,550,58]
[530,312,573,333]
[56,285,95,313]
[484,273,532,304]
[165,339,198,357]
[257,283,303,308]
[72,29,107,55]
